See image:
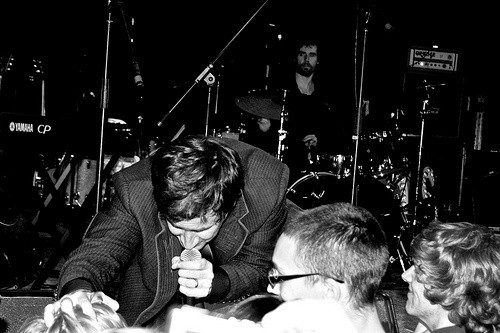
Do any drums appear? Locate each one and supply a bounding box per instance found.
[48,158,96,207]
[286,171,401,255]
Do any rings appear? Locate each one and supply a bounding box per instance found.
[193,278,198,288]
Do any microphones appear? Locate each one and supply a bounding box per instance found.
[131,60,145,88]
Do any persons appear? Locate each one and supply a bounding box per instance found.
[18,304,154,333]
[44,134,289,328]
[401,221,500,333]
[169,202,389,333]
[255,40,336,186]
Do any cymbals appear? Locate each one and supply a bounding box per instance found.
[354,128,421,137]
[416,82,447,91]
[235,97,289,122]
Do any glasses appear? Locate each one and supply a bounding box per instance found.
[266,260,344,289]
